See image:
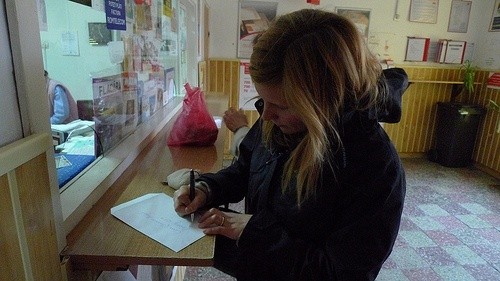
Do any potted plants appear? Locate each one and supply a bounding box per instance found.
[428,59,487,168]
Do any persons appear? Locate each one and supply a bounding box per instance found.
[174,8,406,281]
[43,69,79,125]
[223,30,266,159]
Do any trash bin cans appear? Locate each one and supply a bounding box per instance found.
[427,102,485,169]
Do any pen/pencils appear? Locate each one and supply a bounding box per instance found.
[190,171,196,222]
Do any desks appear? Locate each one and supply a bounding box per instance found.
[60,94,228,281]
[52,120,95,187]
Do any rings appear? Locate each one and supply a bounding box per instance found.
[220,217,224,226]
